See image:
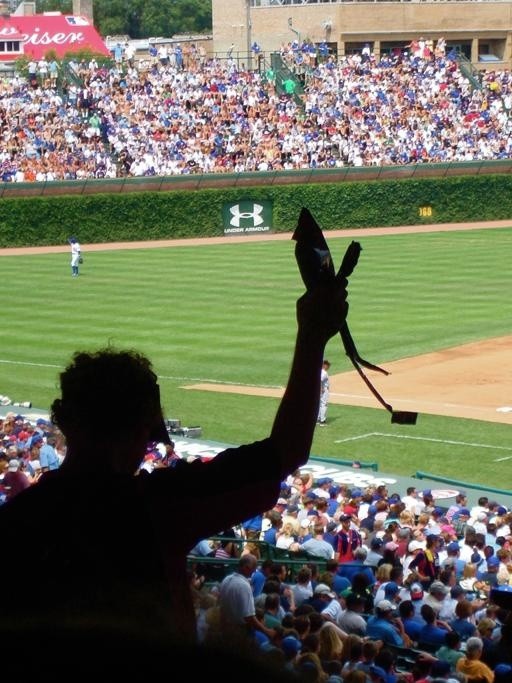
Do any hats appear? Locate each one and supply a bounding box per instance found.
[281,582,512,681]
[277,478,508,567]
[5,415,55,473]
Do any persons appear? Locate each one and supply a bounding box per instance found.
[67,235,85,278]
[0,276,350,683]
[0,411,510,683]
[317,359,332,427]
[2,29,511,183]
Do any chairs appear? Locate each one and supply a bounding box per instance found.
[182,535,508,681]
